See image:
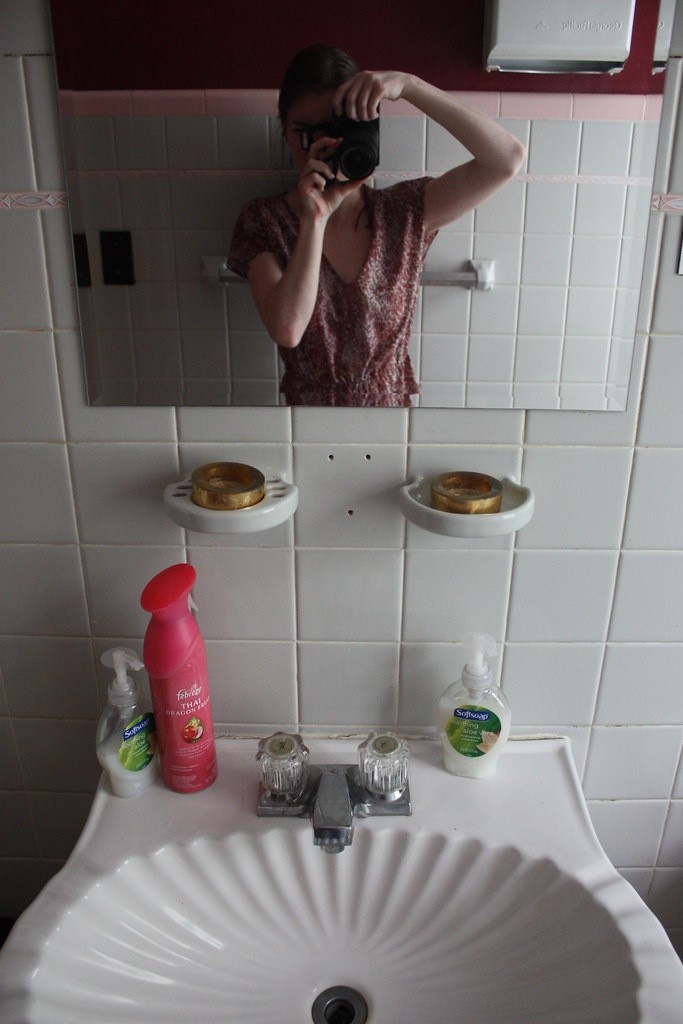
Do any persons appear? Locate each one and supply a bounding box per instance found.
[224,45,528,407]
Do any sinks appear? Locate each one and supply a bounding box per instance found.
[0,736,683,1024]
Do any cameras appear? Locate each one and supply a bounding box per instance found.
[330,96,381,181]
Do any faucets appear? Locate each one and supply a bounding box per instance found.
[254,730,413,856]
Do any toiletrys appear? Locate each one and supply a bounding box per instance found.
[138,562,219,795]
[436,630,513,781]
[94,645,163,799]
